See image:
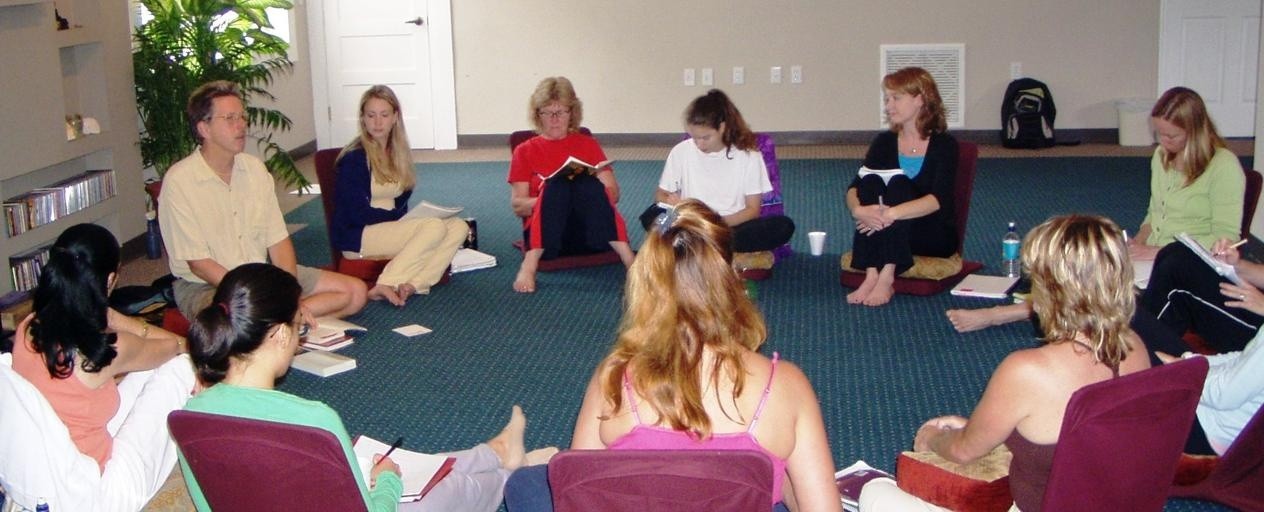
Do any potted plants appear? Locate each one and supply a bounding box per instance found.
[131,1,312,221]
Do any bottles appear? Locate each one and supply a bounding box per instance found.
[147,220,161,259]
[1002,222,1022,281]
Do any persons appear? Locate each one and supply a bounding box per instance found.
[14,224,194,512]
[507,75,636,294]
[1127,237,1264,456]
[641,90,794,253]
[161,81,370,319]
[946,87,1245,332]
[168,262,557,512]
[570,198,848,511]
[858,213,1152,512]
[846,66,959,306]
[333,84,471,308]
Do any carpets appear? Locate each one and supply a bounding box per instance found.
[285,155,1263,511]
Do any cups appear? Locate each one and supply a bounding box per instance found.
[807,231,826,257]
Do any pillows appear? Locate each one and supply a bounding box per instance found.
[895,413,1015,511]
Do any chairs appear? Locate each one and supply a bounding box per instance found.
[167,409,368,512]
[315,147,452,289]
[547,449,775,511]
[1041,356,1210,511]
[0,353,198,512]
[508,126,623,272]
[1167,400,1263,511]
[1134,163,1263,303]
[839,138,976,297]
[682,133,790,282]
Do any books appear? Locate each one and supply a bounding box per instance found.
[289,318,369,380]
[532,152,613,179]
[450,248,497,277]
[352,429,455,505]
[950,274,1022,299]
[835,459,897,512]
[657,202,677,211]
[7,168,119,294]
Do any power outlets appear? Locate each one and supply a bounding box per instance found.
[791,65,800,83]
[731,69,744,86]
[771,66,782,84]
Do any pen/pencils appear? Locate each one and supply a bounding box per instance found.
[1123,228,1128,243]
[1212,238,1246,259]
[537,173,544,180]
[879,197,885,228]
[378,438,402,465]
[676,181,680,197]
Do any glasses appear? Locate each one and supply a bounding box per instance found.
[269,319,310,343]
[195,112,251,126]
[536,107,573,121]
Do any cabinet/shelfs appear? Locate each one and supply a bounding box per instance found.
[1,1,121,299]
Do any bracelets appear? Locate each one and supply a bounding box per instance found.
[140,317,149,339]
[174,336,183,354]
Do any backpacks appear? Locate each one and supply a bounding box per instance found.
[1000,77,1061,151]
